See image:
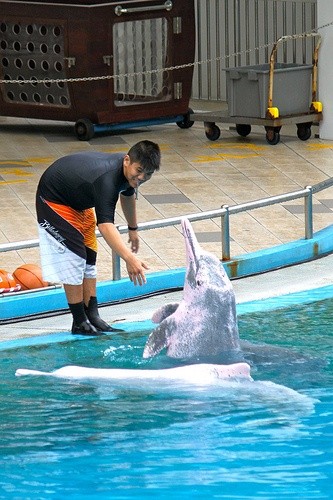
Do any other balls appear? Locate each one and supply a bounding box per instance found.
[11,263,48,291]
[0,269,16,288]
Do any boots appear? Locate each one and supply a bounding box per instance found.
[84,296,113,331]
[68,301,99,336]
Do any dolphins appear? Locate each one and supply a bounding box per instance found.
[142,217,241,358]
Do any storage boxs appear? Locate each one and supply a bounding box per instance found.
[221,61,315,119]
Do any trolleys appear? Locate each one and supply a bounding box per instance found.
[186,31,324,144]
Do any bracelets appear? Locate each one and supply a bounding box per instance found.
[128,225,138,229]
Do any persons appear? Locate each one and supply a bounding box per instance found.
[36,140,161,336]
